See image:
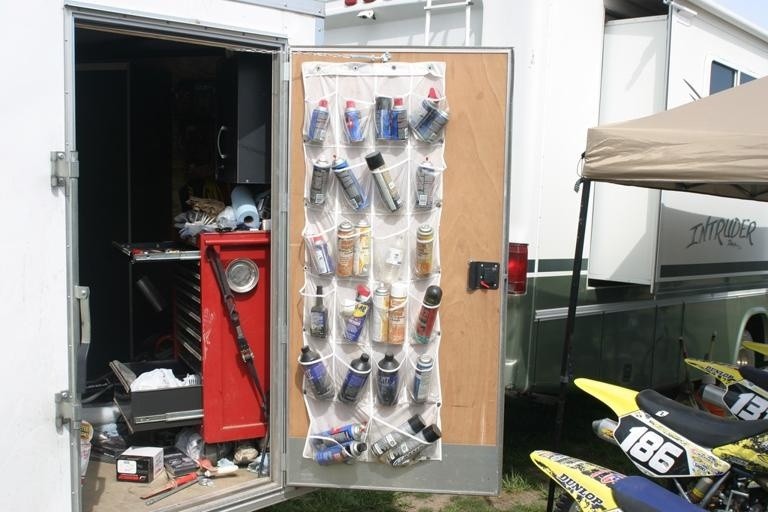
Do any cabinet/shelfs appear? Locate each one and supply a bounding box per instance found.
[216,55,271,186]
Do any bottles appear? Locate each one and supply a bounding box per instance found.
[343,100,364,144]
[307,100,331,141]
[389,97,408,140]
[311,286,329,339]
[374,352,401,406]
[300,346,336,400]
[338,352,370,404]
[313,237,333,274]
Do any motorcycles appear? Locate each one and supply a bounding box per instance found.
[573,376,768,512]
[752,332,768,367]
[527,447,710,512]
[683,356,767,422]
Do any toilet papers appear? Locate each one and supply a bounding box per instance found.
[232,185,260,229]
